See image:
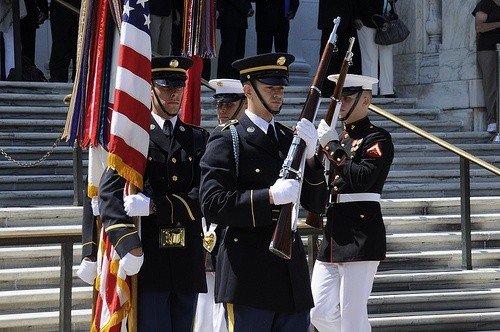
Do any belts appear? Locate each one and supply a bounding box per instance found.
[328,193,381,203]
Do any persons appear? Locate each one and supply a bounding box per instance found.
[0,0,50,81]
[149,0,181,57]
[48,0,84,82]
[96,56,209,332]
[309,72,395,330]
[199,53,328,330]
[216,0,254,79]
[255,0,300,55]
[471,0,500,132]
[79,117,209,285]
[192,76,251,330]
[354,0,400,98]
[318,0,363,96]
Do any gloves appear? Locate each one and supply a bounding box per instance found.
[118,252,145,279]
[123,192,151,217]
[76,258,98,285]
[317,118,340,149]
[295,117,318,160]
[270,177,299,205]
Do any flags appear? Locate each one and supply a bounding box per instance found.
[87,0,154,331]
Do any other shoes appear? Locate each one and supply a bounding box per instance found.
[385,92,399,98]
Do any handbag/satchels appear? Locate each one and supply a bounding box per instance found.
[370,0,410,46]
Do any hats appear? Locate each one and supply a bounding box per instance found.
[231,52,295,86]
[327,73,379,91]
[151,56,194,88]
[208,79,247,103]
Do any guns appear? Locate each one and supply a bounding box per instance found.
[267,15,343,258]
[303,36,357,229]
